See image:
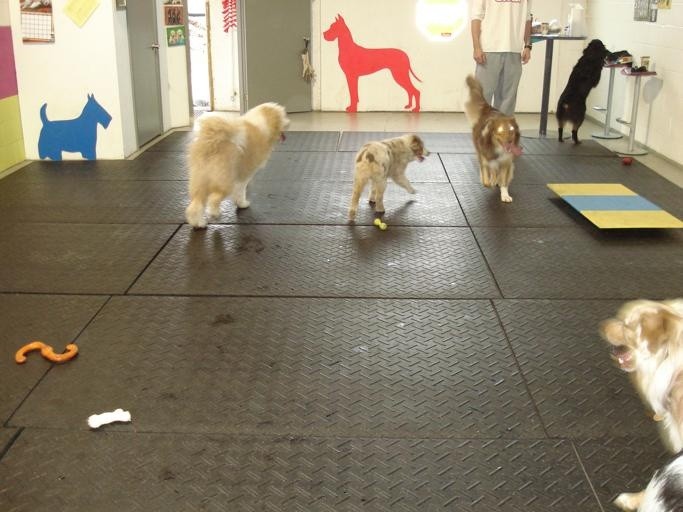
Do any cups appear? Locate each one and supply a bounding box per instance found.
[640,56,649,71]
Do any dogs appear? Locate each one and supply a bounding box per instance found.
[612,454,683,512]
[176,29,184,44]
[555,38,614,145]
[176,100,292,230]
[347,133,431,222]
[463,73,525,203]
[168,31,176,45]
[596,296,683,457]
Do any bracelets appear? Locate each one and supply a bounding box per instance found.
[525,45,532,50]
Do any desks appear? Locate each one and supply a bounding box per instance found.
[520,34,587,141]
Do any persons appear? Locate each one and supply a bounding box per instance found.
[471,0,533,117]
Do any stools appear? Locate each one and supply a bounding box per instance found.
[592,62,632,138]
[616,68,655,155]
